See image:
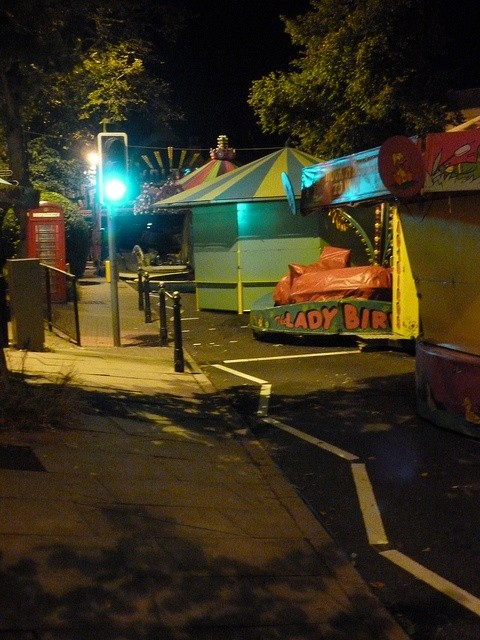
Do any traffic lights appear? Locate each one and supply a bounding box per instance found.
[97,133,130,209]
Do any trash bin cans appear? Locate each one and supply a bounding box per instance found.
[105,261,111,283]
